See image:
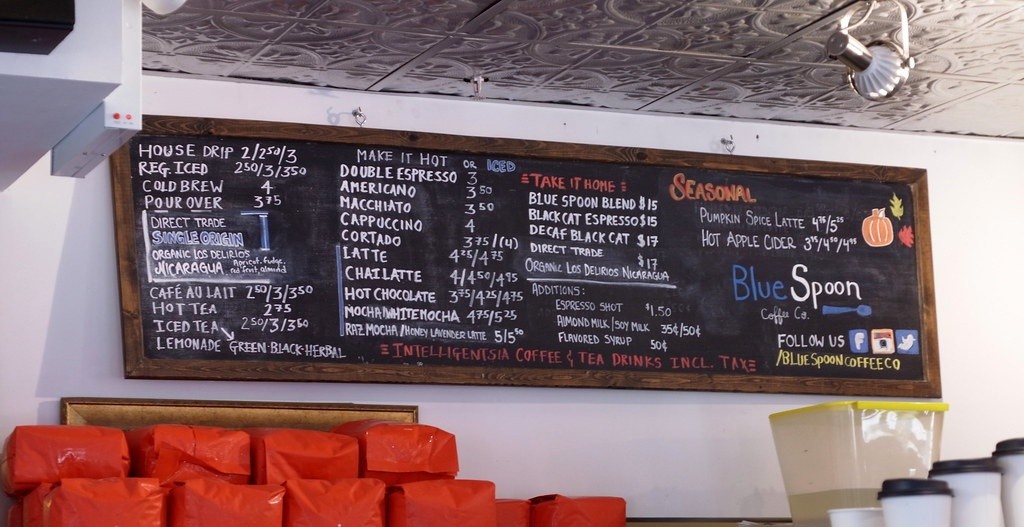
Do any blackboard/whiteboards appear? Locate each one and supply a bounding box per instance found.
[109,115,942,398]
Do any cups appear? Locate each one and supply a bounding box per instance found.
[826,507,882,527]
[926,457,1004,527]
[877,477,955,527]
[991,438,1024,527]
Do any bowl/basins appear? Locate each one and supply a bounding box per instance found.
[767,400,949,527]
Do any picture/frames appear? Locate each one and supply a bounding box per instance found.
[57,395,420,435]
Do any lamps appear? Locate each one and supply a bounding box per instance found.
[823,0,921,104]
